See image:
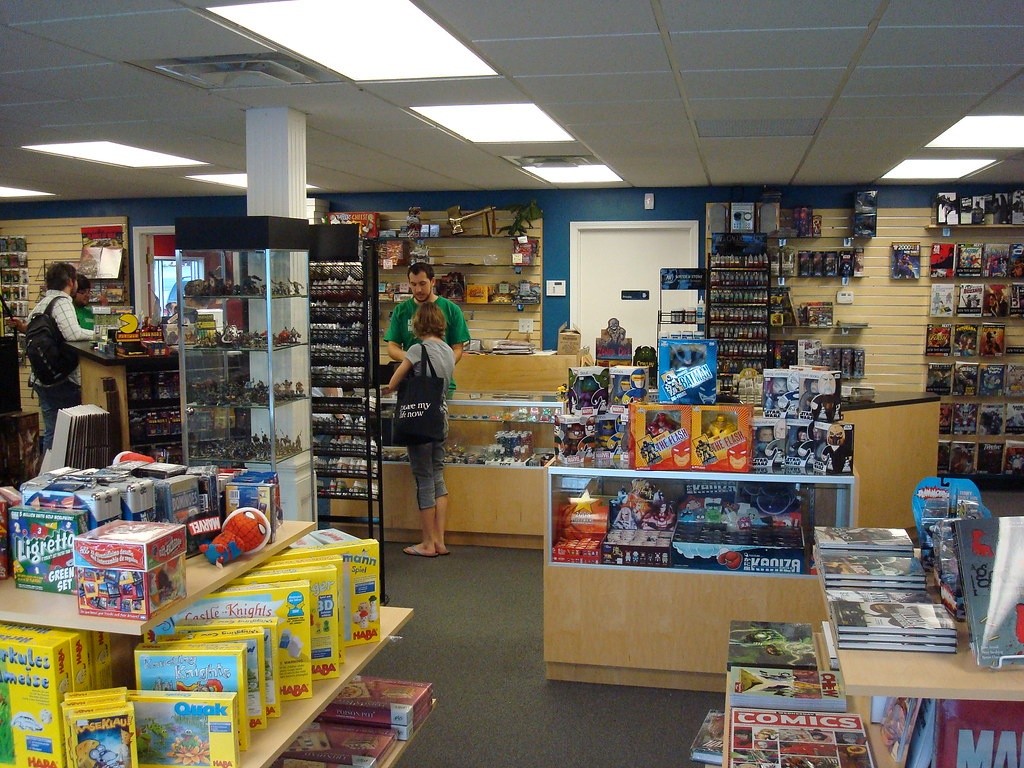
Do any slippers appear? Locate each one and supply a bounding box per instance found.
[402,546,438,557]
[436,550,449,554]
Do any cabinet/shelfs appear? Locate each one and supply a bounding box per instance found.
[0,520,437,768]
[659,267,709,374]
[175,217,313,520]
[708,232,771,374]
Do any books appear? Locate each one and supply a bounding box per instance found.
[953,515,1024,666]
[690,709,724,765]
[727,618,819,667]
[879,696,917,763]
[934,699,1024,768]
[815,526,957,653]
[728,707,875,768]
[728,665,847,712]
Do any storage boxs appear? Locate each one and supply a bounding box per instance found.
[550,336,862,574]
[0,461,432,767]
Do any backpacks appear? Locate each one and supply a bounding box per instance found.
[26,296,79,384]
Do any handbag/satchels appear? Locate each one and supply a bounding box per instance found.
[391,344,444,444]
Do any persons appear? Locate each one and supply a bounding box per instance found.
[183,279,212,313]
[382,261,471,401]
[389,302,456,558]
[8,260,100,449]
